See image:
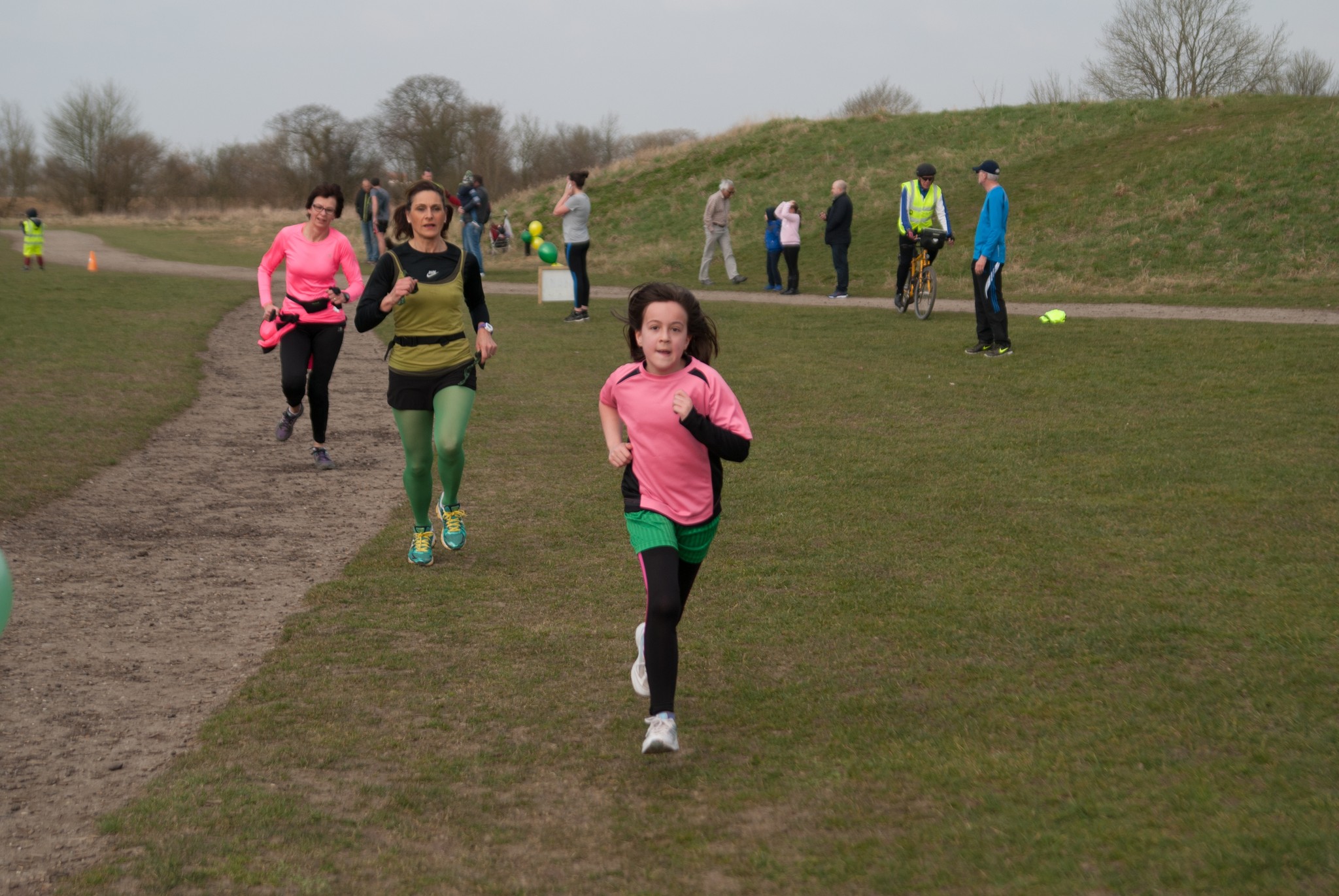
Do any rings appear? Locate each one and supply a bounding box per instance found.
[490,353,493,356]
[334,298,337,301]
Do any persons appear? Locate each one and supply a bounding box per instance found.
[258,183,364,469]
[894,164,955,308]
[349,167,492,277]
[698,178,749,285]
[553,170,591,322]
[354,179,497,568]
[598,283,753,755]
[18,208,47,270]
[763,180,854,298]
[963,159,1013,357]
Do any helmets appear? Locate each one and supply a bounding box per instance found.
[916,162,936,177]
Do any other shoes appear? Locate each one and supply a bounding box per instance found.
[773,285,783,291]
[780,288,799,295]
[471,221,480,228]
[895,294,903,307]
[459,219,464,227]
[765,285,774,289]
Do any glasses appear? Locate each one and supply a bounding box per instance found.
[312,204,335,215]
[976,170,984,173]
[727,190,734,194]
[921,176,933,181]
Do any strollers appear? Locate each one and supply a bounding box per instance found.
[487,209,515,255]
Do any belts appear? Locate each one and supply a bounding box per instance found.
[714,223,725,228]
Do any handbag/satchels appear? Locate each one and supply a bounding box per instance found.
[920,228,947,249]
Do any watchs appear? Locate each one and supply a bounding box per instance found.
[343,291,351,304]
[477,322,493,332]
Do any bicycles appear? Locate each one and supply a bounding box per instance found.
[897,227,950,320]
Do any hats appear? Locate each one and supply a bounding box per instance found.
[461,170,474,184]
[972,160,1000,175]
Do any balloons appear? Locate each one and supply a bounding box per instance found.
[521,220,563,266]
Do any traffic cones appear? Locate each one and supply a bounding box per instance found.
[87,251,99,272]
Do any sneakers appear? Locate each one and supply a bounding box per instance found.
[702,279,714,285]
[829,291,848,298]
[734,276,747,283]
[310,447,334,469]
[984,344,1012,357]
[408,519,436,567]
[275,403,304,440]
[564,311,584,322]
[965,343,994,354]
[436,491,467,550]
[582,310,589,319]
[631,622,649,696]
[641,712,679,753]
